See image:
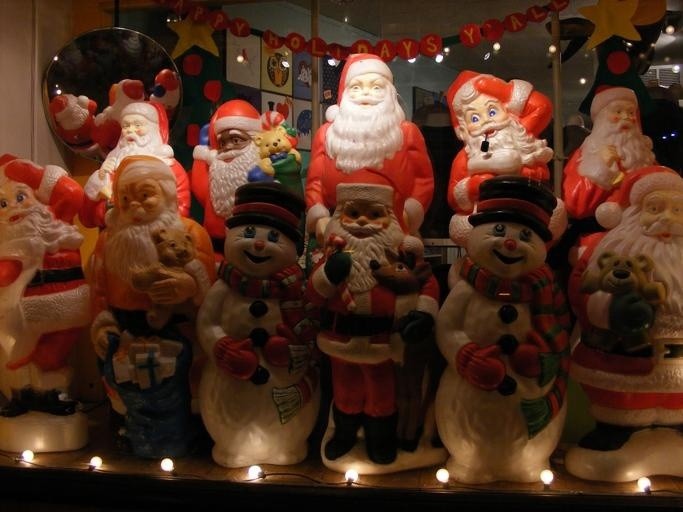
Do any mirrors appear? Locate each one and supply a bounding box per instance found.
[42,25,184,166]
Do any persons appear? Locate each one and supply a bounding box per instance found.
[0,48,682,492]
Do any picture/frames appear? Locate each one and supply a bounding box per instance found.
[225,28,324,151]
[412,86,436,116]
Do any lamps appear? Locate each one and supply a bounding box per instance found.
[661,15,679,34]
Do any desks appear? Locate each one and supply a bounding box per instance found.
[0,444,683,512]
